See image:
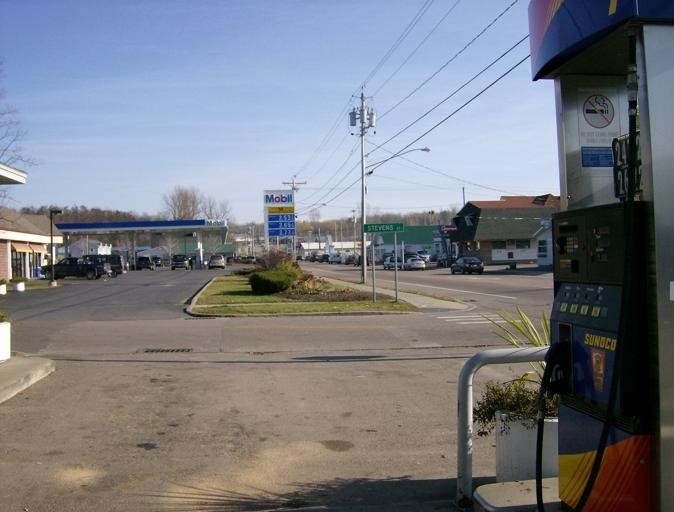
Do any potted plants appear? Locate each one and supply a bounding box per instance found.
[9,277,29,292]
[475,304,559,482]
[0,278,7,295]
[0,309,14,360]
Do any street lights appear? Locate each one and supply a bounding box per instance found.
[350,209,358,255]
[47,208,65,287]
[360,145,433,285]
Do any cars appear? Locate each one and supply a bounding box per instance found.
[450,256,486,275]
[234,252,432,272]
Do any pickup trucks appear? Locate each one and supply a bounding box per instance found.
[39,256,113,281]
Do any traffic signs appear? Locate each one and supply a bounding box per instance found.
[362,222,405,233]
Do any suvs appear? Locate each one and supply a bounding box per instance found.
[208,254,225,269]
[170,254,192,270]
[130,255,156,271]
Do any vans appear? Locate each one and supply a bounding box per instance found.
[80,254,126,279]
[152,256,161,266]
